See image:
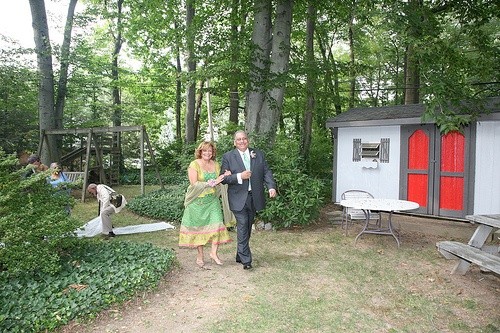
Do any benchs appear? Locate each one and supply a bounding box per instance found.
[64,172,85,189]
[436,241,500,278]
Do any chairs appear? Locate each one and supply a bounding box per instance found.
[341,190,382,235]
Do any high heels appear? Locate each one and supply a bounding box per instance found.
[195,252,225,272]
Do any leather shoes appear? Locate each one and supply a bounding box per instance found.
[236,257,253,270]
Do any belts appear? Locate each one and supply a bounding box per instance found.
[248,190,252,194]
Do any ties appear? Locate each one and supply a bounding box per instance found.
[97,200,102,217]
[243,153,252,191]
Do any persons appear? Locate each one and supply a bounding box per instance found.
[88,183,126,239]
[178,142,231,269]
[25,155,47,177]
[45,162,73,215]
[12,148,36,180]
[220,130,276,270]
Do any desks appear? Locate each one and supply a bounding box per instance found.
[340,199,420,248]
[465,215,500,249]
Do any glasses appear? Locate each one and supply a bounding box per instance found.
[236,137,249,142]
[88,189,93,193]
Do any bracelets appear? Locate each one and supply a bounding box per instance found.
[208,179,216,187]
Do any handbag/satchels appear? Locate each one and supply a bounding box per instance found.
[109,192,123,208]
